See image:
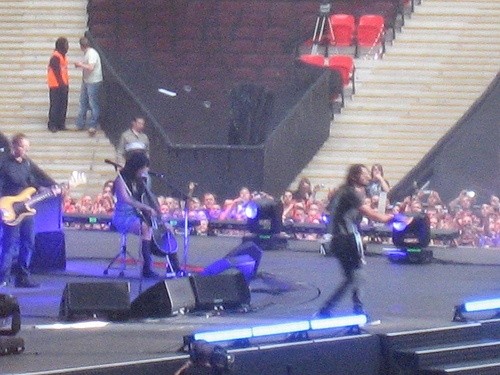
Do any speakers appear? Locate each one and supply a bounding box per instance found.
[190,273,251,313]
[35,231,66,273]
[57,282,132,320]
[199,254,257,279]
[225,241,263,275]
[131,278,196,319]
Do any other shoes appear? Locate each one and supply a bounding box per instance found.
[15,276,40,288]
[357,313,381,326]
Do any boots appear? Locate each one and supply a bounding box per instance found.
[167,252,186,277]
[141,240,159,277]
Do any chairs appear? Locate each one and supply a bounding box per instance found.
[87,0,411,109]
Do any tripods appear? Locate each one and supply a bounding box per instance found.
[159,177,197,279]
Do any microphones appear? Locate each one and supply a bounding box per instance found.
[148,171,165,177]
[104,159,122,168]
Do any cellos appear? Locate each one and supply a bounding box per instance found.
[139,176,178,280]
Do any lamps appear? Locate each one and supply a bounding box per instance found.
[453,297,500,322]
[179,314,370,352]
[245,197,287,249]
[388,212,433,264]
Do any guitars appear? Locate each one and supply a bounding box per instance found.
[0,170,88,226]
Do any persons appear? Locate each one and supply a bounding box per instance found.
[0,132,64,288]
[312,164,413,326]
[60,179,118,230]
[361,163,500,249]
[111,152,191,279]
[158,177,336,240]
[71,37,103,133]
[121,115,151,171]
[48,38,70,131]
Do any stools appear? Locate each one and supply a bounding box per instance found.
[103,223,143,277]
